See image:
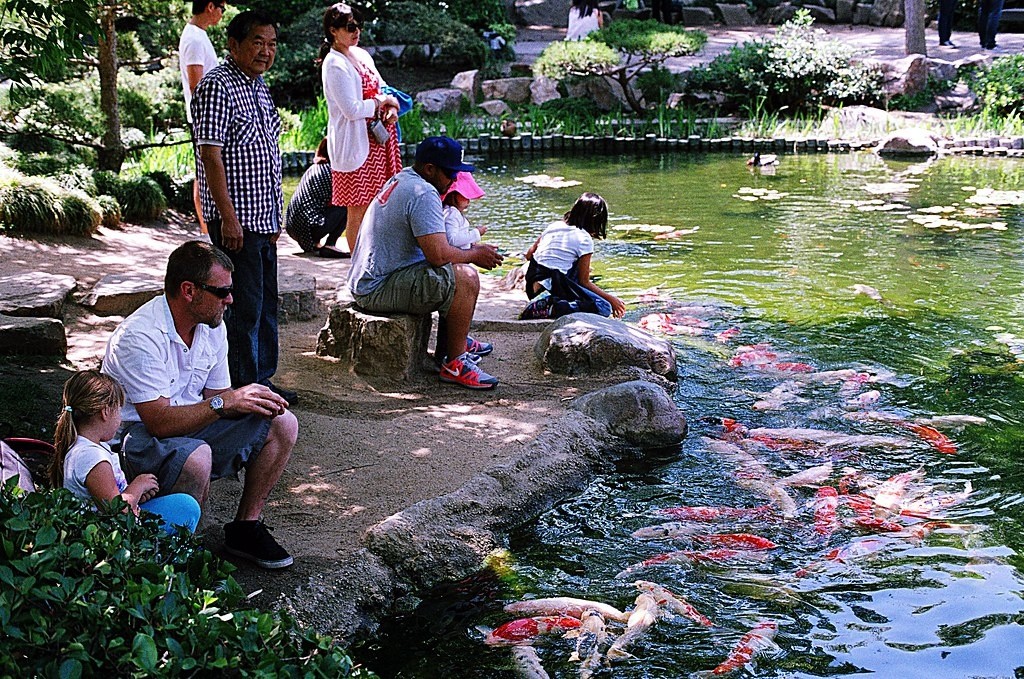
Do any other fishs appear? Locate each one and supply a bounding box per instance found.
[689,621,782,679]
[510,646,550,679]
[635,581,715,627]
[604,591,677,662]
[504,596,632,623]
[563,607,628,679]
[614,224,986,582]
[477,615,581,646]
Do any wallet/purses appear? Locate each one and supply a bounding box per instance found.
[371,120,391,144]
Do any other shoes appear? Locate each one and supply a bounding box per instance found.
[267,383,299,406]
[304,246,351,259]
[467,351,481,364]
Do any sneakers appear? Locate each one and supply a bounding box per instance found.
[517,290,552,320]
[466,336,493,355]
[440,351,499,388]
[222,519,293,569]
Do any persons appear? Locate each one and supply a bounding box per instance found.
[344,136,504,388]
[178,0,225,244]
[310,2,401,259]
[99,240,298,566]
[566,0,604,42]
[978,0,1009,52]
[285,136,351,259]
[190,10,298,406]
[437,171,488,367]
[937,0,955,46]
[53,368,202,543]
[517,192,626,319]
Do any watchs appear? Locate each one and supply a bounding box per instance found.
[209,394,229,417]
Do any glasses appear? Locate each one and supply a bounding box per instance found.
[192,281,233,299]
[210,0,226,14]
[343,23,363,33]
[435,165,458,180]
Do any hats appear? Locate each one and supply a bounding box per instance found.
[440,171,486,202]
[415,135,476,173]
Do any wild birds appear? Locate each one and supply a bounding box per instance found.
[500,118,517,137]
[747,151,777,167]
